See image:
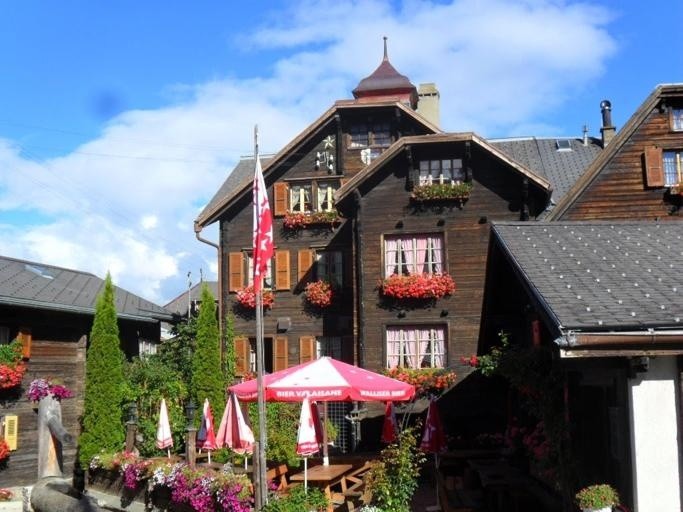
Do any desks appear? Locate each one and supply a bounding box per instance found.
[289,463,354,512]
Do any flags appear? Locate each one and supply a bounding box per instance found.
[253,154,274,296]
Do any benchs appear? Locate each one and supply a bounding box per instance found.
[264,464,302,499]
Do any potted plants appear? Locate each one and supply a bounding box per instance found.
[576,483,621,512]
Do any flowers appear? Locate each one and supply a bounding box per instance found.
[380,271,457,302]
[379,364,456,393]
[282,209,338,228]
[235,284,274,309]
[90,447,254,512]
[302,278,335,308]
[0,343,74,402]
